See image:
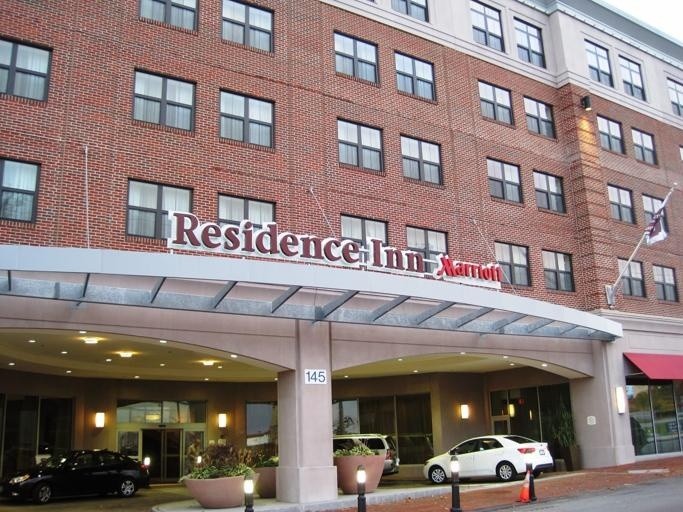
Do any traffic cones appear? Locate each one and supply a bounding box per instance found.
[515,471,530,503]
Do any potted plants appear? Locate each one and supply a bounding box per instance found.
[177,438,386,509]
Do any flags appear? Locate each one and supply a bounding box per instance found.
[643,205,667,246]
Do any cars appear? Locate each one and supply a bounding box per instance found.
[1,448,149,505]
[422,434,554,485]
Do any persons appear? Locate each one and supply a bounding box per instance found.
[186,437,203,474]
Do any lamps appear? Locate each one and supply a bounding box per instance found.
[616,387,626,414]
[580,96,591,110]
[218,410,226,431]
[95,408,105,433]
[460,402,469,422]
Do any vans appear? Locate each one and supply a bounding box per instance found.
[264,433,400,477]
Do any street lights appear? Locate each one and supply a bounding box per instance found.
[243,476,254,512]
[450,455,461,512]
[355,465,366,512]
[523,448,537,502]
